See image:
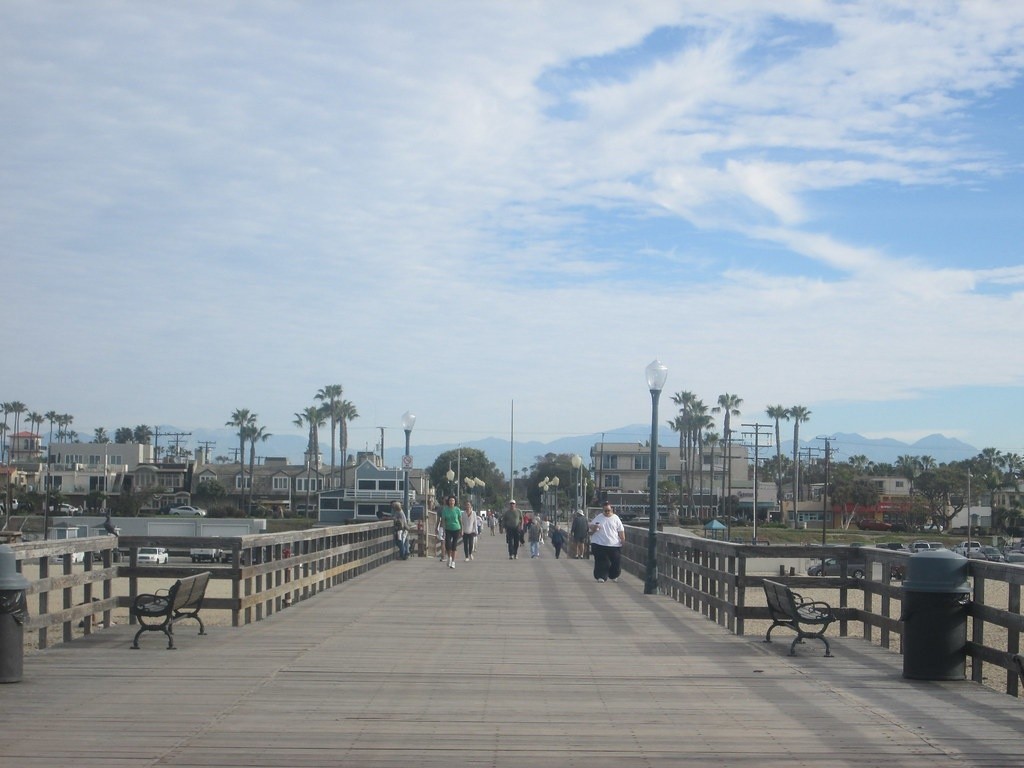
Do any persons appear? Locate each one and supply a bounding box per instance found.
[589,500,625,583]
[381,499,410,560]
[462,499,478,562]
[503,499,523,560]
[571,510,589,559]
[434,495,464,569]
[472,514,549,560]
[527,519,542,560]
[551,525,565,559]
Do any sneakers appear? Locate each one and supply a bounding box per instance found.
[447,558,451,567]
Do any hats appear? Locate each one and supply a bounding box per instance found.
[509,499,516,503]
[577,510,585,515]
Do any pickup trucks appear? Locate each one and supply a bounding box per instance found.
[0,499,32,513]
[955,541,981,557]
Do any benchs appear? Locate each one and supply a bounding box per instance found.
[129,570,213,650]
[761,578,838,657]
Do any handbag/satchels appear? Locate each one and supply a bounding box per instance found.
[539,526,544,545]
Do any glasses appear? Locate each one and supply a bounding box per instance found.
[510,503,516,505]
[603,510,611,512]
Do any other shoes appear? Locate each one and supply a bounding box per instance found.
[509,556,518,559]
[440,556,447,562]
[451,562,455,569]
[464,558,469,562]
[397,554,408,560]
[576,556,578,559]
[607,577,618,583]
[580,555,583,559]
[531,554,539,559]
[597,578,604,583]
[469,553,473,560]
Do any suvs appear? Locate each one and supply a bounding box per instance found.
[190,547,222,563]
[160,502,185,515]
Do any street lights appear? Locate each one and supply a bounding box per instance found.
[464,476,485,514]
[400,410,417,555]
[449,458,467,496]
[105,440,113,508]
[644,356,668,595]
[572,454,582,518]
[538,475,553,538]
[553,476,559,527]
[967,474,974,558]
[447,470,455,496]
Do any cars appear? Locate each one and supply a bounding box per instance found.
[890,564,904,579]
[717,515,738,521]
[221,550,244,562]
[137,547,168,564]
[979,545,1004,562]
[49,503,78,513]
[859,519,893,531]
[169,506,207,517]
[52,551,84,564]
[891,522,944,532]
[1001,542,1024,563]
[966,552,988,560]
[875,541,947,551]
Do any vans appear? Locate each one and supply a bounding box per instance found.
[807,558,865,579]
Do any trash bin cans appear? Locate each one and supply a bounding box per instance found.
[0,545,29,684]
[900,549,970,681]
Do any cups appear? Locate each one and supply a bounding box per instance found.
[595,522,600,530]
